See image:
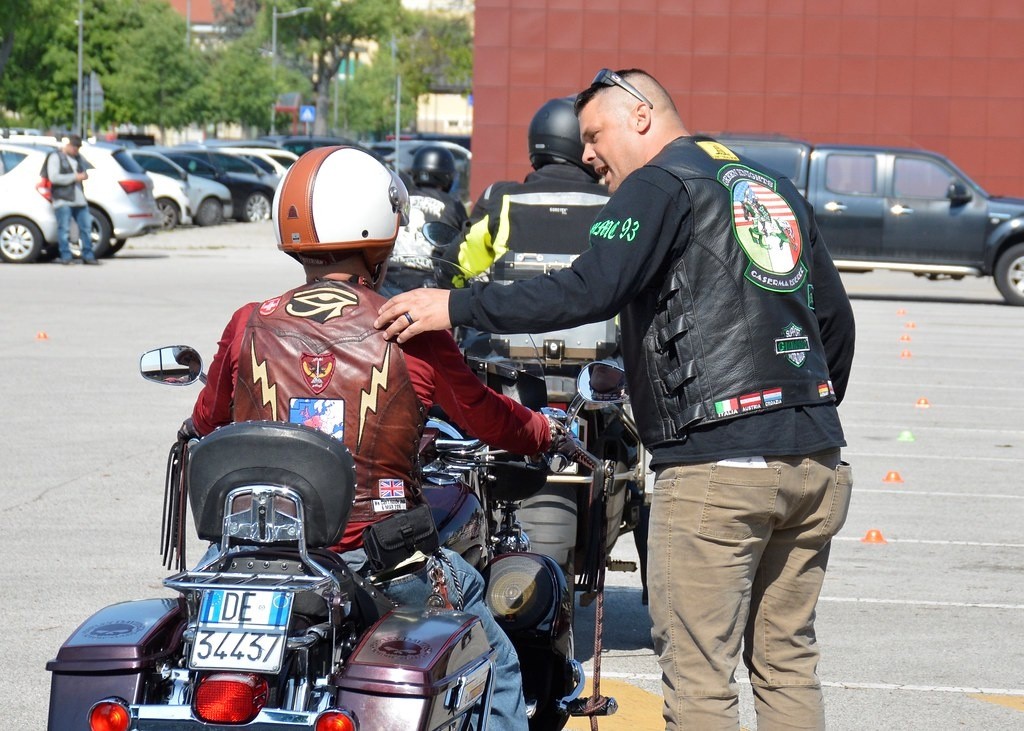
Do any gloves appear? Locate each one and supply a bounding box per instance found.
[177,417,202,444]
[545,414,579,462]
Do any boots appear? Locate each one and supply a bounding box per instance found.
[631,501,651,606]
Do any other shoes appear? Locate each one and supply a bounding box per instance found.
[62,259,75,265]
[528,698,570,731]
[83,259,100,265]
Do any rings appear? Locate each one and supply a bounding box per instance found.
[405,312,413,324]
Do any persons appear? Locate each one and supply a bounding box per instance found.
[440,95,650,607]
[390,147,467,265]
[375,67,856,731]
[193,146,574,731]
[47,135,99,265]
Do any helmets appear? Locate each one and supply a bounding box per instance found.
[529,94,603,181]
[172,347,199,365]
[412,145,455,192]
[272,146,410,253]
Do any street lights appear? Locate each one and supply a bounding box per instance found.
[263,5,313,136]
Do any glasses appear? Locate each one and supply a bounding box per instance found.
[590,68,653,110]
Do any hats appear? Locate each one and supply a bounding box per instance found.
[70,135,82,146]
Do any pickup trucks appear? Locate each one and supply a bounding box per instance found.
[699,130,1024,307]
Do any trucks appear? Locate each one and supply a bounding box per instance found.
[416,88,471,147]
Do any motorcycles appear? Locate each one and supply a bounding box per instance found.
[420,220,648,622]
[44,254,630,731]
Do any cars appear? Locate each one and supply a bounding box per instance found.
[0,129,470,262]
